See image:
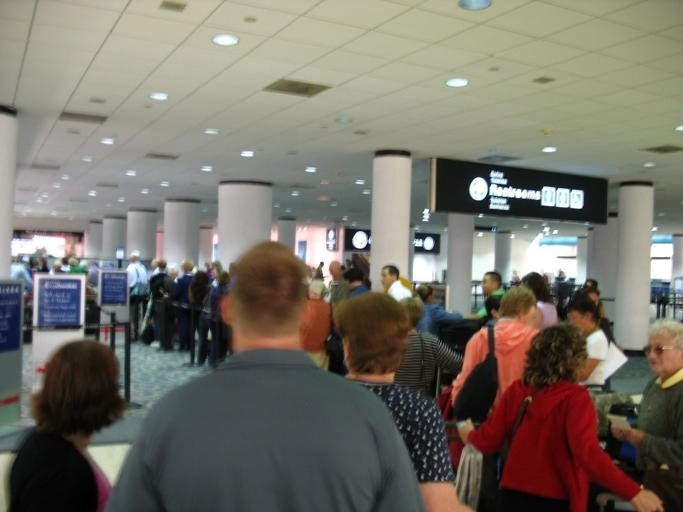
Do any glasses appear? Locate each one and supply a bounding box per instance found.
[643,345,674,354]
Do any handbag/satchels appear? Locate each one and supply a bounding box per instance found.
[584,383,642,464]
[129,284,151,303]
[576,341,627,385]
[324,333,343,358]
[142,326,155,345]
[454,354,498,421]
